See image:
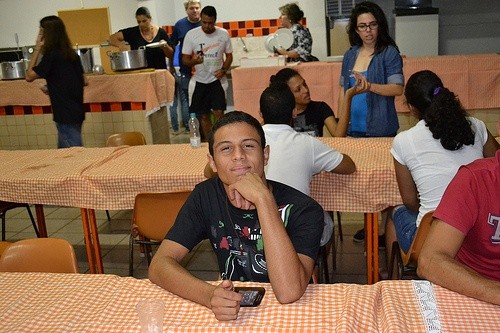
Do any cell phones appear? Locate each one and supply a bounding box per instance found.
[197,51,203,56]
[230,287,265,307]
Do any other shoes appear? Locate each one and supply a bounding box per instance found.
[353,229,365,241]
[172,129,178,134]
[186,127,190,134]
[378,234,388,249]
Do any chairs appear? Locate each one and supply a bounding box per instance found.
[388,210,434,279]
[0,201,40,240]
[105,132,146,221]
[0,236,80,273]
[128,190,192,276]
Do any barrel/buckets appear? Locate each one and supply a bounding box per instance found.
[72,48,91,72]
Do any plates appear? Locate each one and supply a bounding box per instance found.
[264,33,276,51]
[146,41,167,48]
[324,56,344,63]
[273,27,294,50]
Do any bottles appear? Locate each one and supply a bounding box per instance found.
[188,113,200,149]
[92,65,104,75]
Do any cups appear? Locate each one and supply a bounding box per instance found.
[135,298,165,333]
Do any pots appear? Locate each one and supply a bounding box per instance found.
[0,59,31,80]
[106,45,148,71]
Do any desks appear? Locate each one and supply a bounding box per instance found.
[0,137,404,284]
[0,270,500,333]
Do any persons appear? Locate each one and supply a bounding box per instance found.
[181,5,233,142]
[270,68,371,137]
[109,7,173,69]
[417,147,500,306]
[148,111,324,321]
[204,81,356,285]
[169,42,191,135]
[337,1,404,249]
[169,0,205,143]
[274,3,313,62]
[384,69,495,280]
[27,15,90,149]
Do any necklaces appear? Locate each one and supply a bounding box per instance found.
[141,27,154,41]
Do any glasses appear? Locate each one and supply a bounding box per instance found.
[355,20,379,32]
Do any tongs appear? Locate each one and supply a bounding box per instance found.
[238,36,248,52]
